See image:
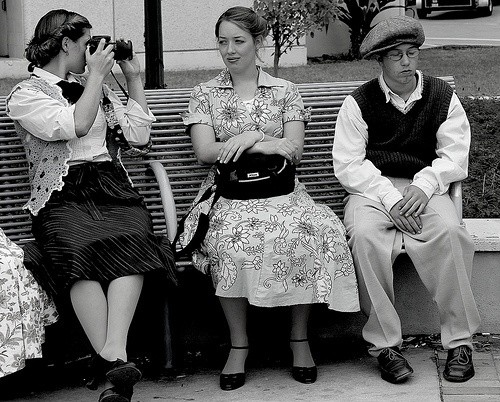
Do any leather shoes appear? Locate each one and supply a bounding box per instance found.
[376,346,413,382]
[442,345,475,382]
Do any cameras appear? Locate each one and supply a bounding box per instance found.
[90,35,132,61]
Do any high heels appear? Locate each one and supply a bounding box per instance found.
[220,342,250,391]
[99,387,129,402]
[86,353,142,390]
[288,338,318,384]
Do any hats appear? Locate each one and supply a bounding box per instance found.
[359,15,426,61]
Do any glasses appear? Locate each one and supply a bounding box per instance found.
[382,46,421,61]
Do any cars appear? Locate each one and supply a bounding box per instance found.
[416,0,494,19]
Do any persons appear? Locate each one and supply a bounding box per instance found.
[170,5,361,391]
[6,8,178,402]
[332,15,481,382]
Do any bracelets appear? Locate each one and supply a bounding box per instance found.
[260,131,264,141]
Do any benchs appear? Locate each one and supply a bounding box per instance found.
[0,75,463,370]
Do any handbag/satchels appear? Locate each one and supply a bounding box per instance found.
[215,151,294,199]
